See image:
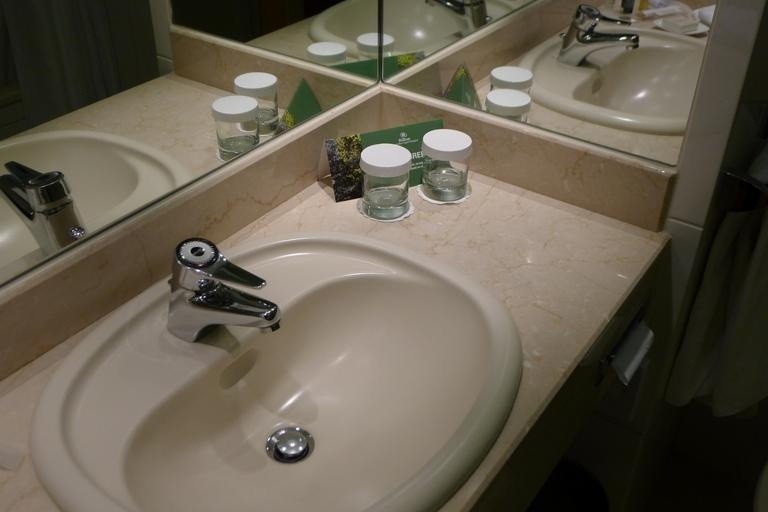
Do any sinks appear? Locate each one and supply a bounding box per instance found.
[0,131,200,289]
[29,229,523,510]
[307,0,517,60]
[518,27,707,135]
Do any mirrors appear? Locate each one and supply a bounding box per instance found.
[382,0,534,82]
[382,0,717,167]
[0,0,382,284]
[170,0,378,81]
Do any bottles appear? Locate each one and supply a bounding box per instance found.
[305,31,395,67]
[485,65,534,122]
[359,142,412,220]
[234,71,279,136]
[209,94,259,160]
[421,127,473,203]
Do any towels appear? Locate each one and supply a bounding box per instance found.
[659,205,768,417]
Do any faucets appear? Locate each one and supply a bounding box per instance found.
[167,237,282,345]
[423,0,491,37]
[0,160,87,256]
[558,4,640,65]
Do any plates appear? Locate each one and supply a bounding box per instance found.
[654,17,709,36]
